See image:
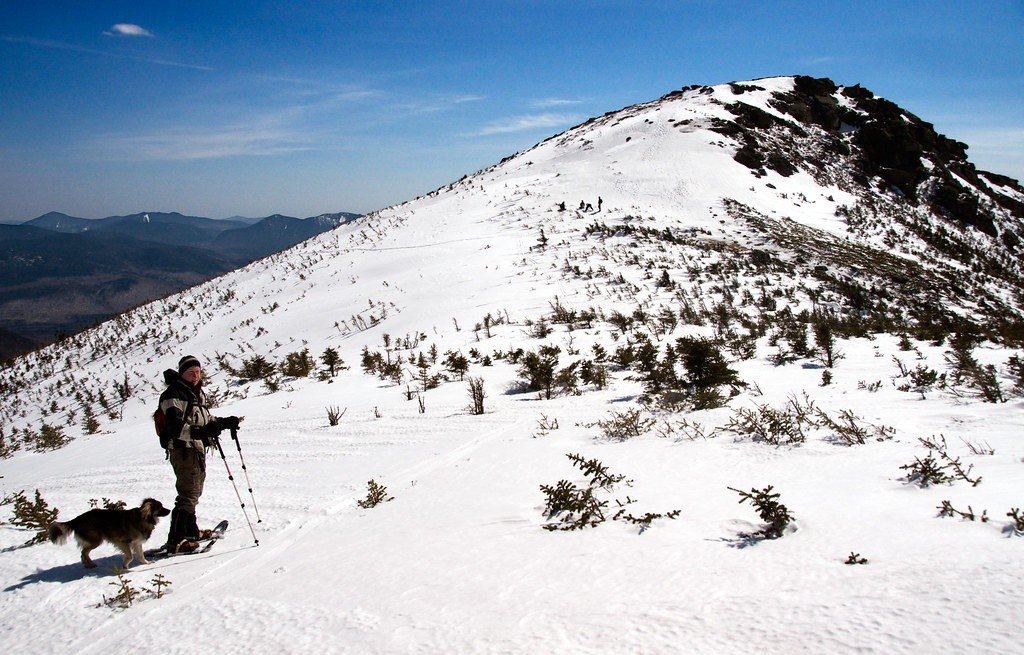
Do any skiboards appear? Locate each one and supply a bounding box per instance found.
[141,520,228,555]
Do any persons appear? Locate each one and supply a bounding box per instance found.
[580,196,602,213]
[159,355,239,552]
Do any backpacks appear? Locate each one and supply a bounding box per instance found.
[153,382,194,448]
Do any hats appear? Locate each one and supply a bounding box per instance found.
[179,355,200,376]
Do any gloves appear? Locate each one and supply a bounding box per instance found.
[216,416,239,430]
[190,421,221,440]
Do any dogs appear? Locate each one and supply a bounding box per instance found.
[48,497,171,570]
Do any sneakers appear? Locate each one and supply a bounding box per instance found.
[167,532,199,553]
[184,529,211,542]
